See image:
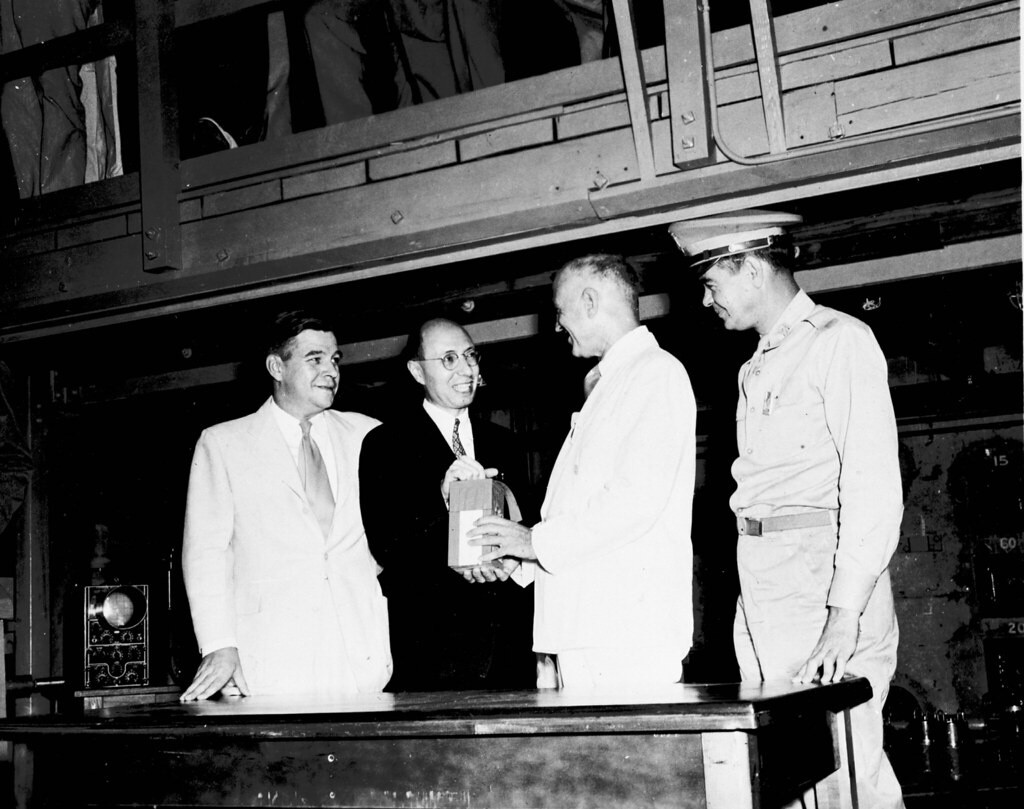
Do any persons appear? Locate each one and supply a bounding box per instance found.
[178,309,385,703]
[666,211,906,809]
[359,316,538,693]
[461,254,697,693]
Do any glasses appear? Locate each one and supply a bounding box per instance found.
[420,348,481,370]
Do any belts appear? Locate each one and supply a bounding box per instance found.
[737,510,832,536]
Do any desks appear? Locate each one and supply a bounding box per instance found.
[0,677,872,809]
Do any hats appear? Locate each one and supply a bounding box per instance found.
[668,208,803,280]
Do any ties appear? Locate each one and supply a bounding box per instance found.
[300,421,336,540]
[452,419,466,458]
[742,337,769,396]
[583,364,601,399]
